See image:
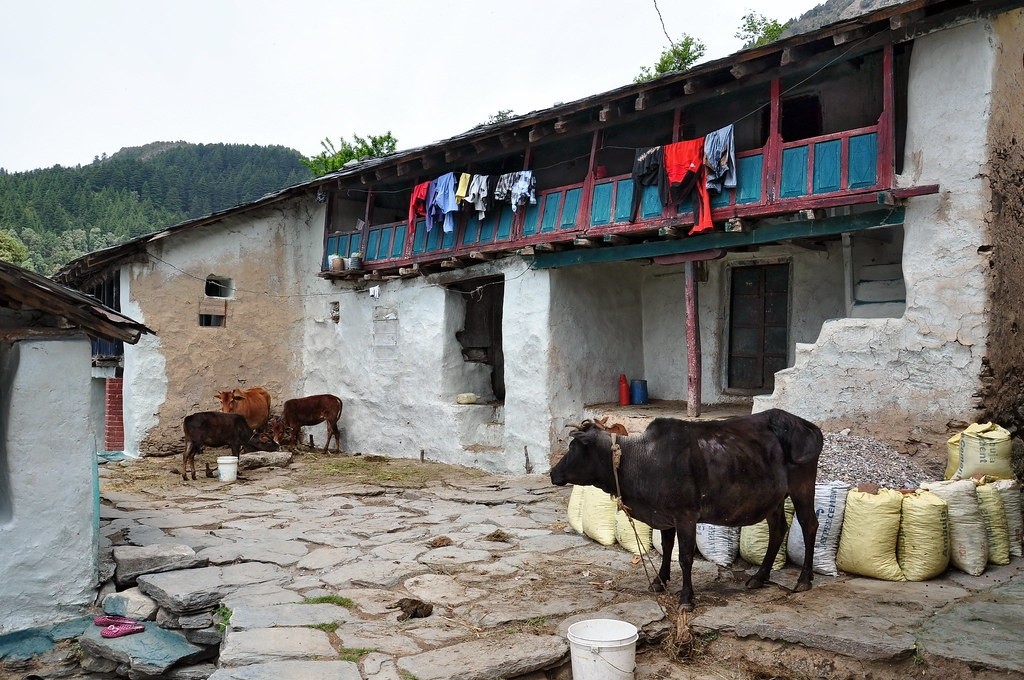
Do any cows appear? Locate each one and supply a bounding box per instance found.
[181,411,279,485]
[214,386,271,429]
[271,394,343,454]
[548,408,824,608]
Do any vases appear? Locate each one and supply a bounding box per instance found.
[328,253,365,272]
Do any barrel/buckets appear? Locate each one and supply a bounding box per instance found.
[630,379,648,404]
[567,619,639,680]
[217,456,238,482]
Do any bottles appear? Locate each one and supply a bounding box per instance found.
[620,373,629,406]
